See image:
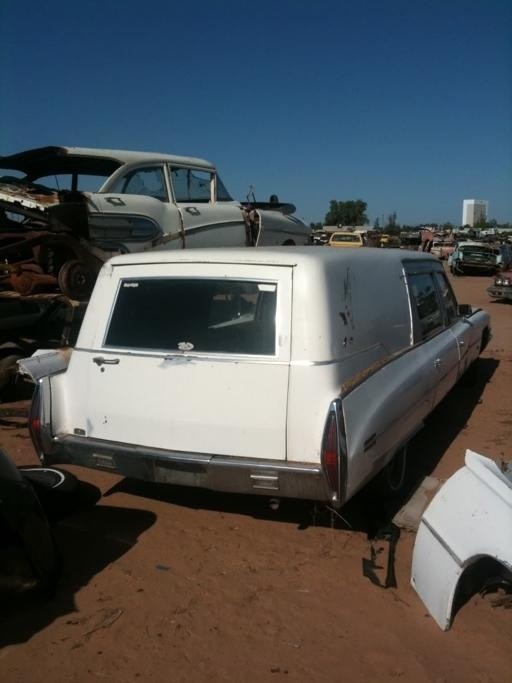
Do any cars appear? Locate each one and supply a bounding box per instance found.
[17,244,493,507]
[0,143,315,394]
[314,224,512,303]
